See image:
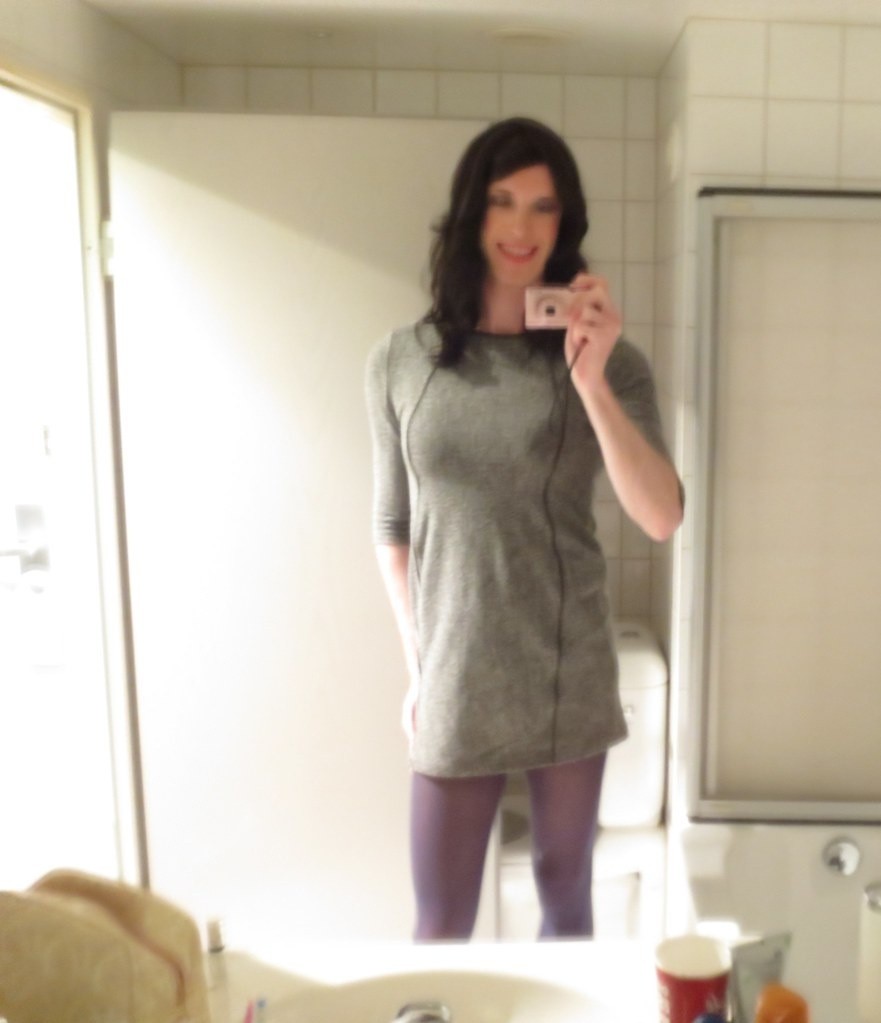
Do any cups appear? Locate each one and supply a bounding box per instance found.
[654,934,732,1023]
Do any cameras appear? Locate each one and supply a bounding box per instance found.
[523,285,575,331]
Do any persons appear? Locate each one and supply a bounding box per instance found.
[368,120,685,944]
